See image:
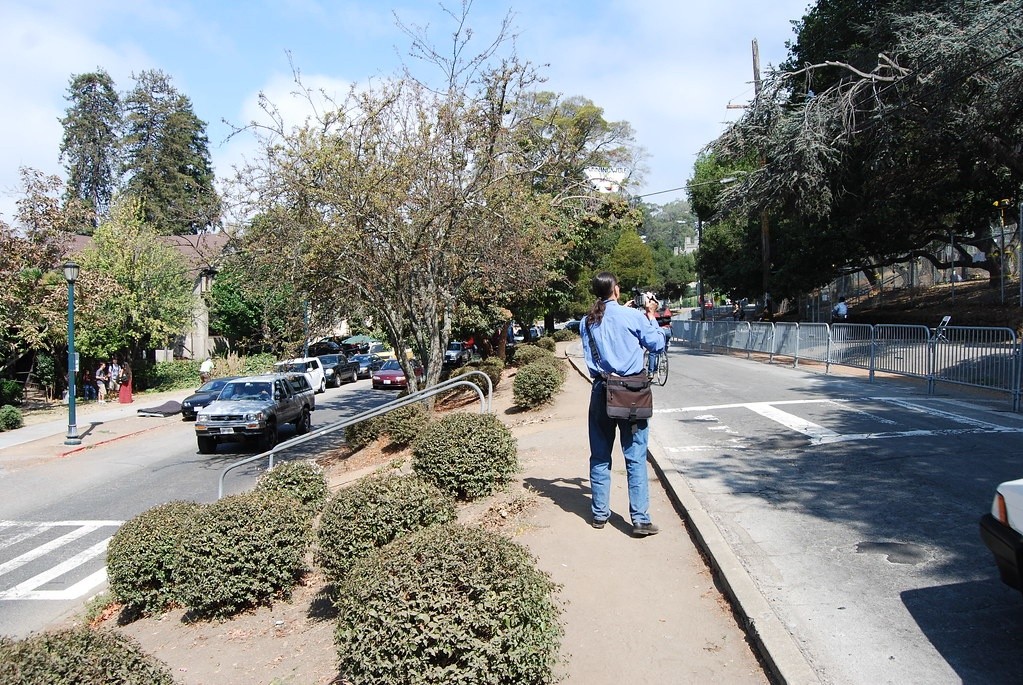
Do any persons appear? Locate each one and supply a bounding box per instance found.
[580,271,672,535]
[82,356,134,404]
[200,357,214,385]
[833,296,848,318]
[230,385,248,401]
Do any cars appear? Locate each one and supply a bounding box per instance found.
[562,320,580,335]
[180,374,252,421]
[272,323,544,394]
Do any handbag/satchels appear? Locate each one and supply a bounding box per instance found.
[601,370,653,420]
[116,369,128,384]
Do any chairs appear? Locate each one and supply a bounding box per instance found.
[930,316,952,343]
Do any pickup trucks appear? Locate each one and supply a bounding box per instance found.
[193,373,316,455]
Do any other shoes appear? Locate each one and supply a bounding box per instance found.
[646,372,654,378]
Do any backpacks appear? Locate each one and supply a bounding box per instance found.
[655,307,672,325]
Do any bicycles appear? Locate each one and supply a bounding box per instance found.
[643,348,669,387]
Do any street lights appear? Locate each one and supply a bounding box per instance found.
[62,260,83,445]
[993,199,1010,305]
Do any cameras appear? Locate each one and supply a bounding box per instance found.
[632,285,648,307]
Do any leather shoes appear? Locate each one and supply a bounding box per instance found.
[592,520,606,528]
[633,523,658,535]
